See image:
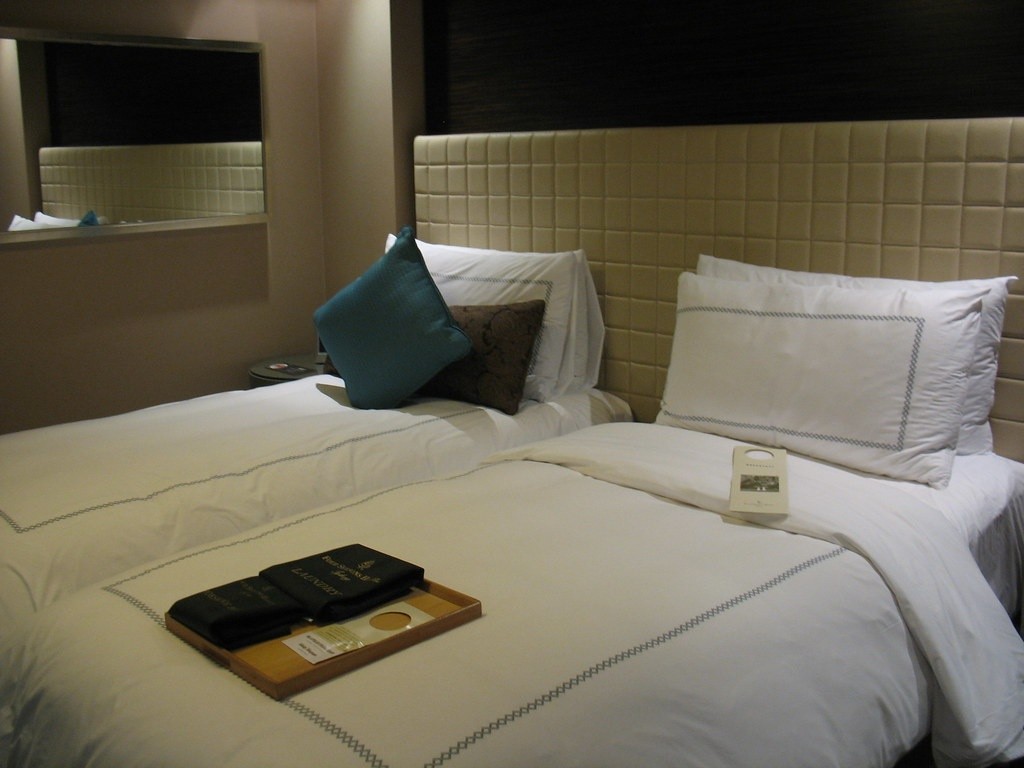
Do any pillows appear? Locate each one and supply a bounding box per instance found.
[657,253,1020,489]
[8,209,147,231]
[313,224,606,418]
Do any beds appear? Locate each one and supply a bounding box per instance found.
[0,370,1024,768]
